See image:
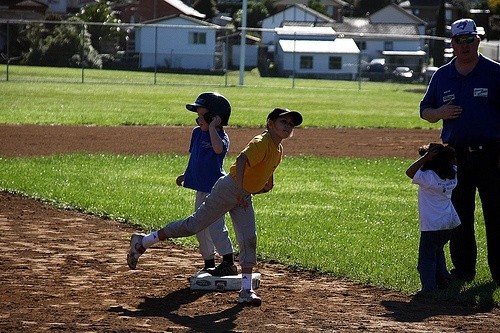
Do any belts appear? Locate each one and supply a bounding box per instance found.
[458,143,492,152]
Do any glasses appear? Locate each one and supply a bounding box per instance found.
[454,36,476,44]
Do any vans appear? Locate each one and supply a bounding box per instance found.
[366,58,388,82]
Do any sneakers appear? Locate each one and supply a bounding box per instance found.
[187,268,216,281]
[207,261,238,275]
[128,234,146,269]
[238,289,261,307]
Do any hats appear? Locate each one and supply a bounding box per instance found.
[451,19,477,36]
[267,108,303,126]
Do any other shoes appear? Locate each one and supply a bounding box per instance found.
[417,290,436,297]
[448,269,475,278]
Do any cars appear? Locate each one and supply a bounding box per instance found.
[392,66,414,82]
[422,66,439,84]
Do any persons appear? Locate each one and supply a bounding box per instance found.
[420,18,500,287]
[127,108,303,305]
[405,142,461,298]
[176,92,238,276]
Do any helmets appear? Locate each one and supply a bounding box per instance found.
[186,93,231,126]
